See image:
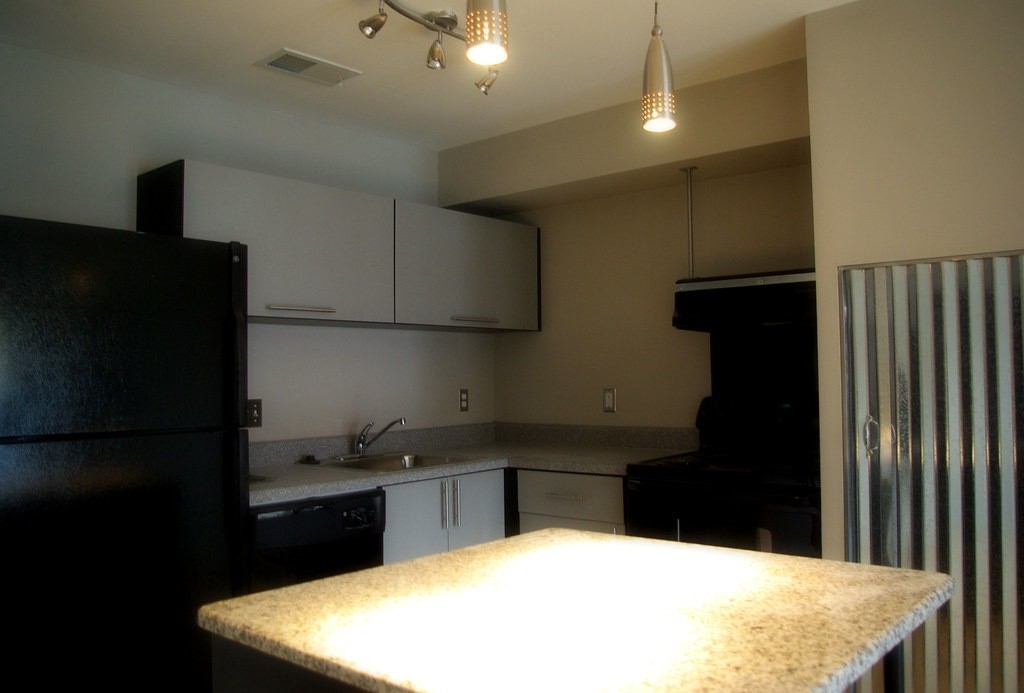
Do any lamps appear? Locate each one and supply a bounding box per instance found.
[637,1,678,135]
[465,1,513,70]
[358,0,500,97]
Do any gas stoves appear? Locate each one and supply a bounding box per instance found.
[624,429,821,487]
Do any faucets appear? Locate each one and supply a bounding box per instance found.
[354,417,406,458]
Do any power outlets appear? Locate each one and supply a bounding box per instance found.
[602,387,617,413]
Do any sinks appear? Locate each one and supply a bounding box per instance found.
[318,451,490,473]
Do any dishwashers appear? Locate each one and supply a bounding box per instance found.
[247,487,386,596]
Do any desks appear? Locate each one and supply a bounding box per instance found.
[197,525,958,693]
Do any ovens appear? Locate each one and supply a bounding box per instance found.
[625,480,822,560]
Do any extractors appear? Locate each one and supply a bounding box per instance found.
[670,271,817,333]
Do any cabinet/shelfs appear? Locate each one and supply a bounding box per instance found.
[134,156,543,334]
[373,468,506,567]
[511,470,632,538]
